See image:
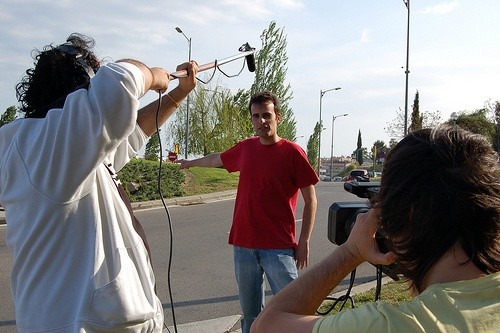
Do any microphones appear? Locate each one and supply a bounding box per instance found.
[245,43,255,72]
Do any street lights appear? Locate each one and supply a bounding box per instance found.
[330,113,348,182]
[175,26,191,158]
[317,87,342,177]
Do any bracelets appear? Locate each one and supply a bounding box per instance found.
[165,93,180,108]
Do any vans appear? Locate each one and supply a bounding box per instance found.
[350,170,368,183]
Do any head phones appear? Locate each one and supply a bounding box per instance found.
[56,44,96,81]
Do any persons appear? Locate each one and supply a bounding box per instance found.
[249,123,500,333]
[174,92,320,333]
[0,32,199,333]
